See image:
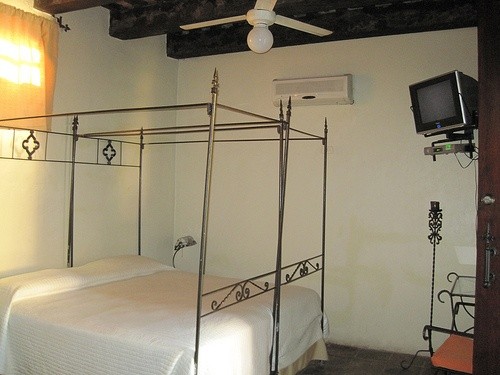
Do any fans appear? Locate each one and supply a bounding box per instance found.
[179,0,333,37]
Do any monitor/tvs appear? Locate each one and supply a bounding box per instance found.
[408,69,481,136]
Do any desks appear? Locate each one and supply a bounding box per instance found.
[439,272,476,335]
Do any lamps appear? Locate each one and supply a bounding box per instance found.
[247,24,273,53]
[173,235,197,268]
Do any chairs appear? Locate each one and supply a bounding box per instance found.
[422,301,475,375]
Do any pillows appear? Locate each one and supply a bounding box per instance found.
[0,254,177,302]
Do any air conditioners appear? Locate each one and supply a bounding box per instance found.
[272,74,355,107]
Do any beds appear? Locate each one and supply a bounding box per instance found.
[0,69,327,375]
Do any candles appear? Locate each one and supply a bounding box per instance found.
[432,200,439,209]
